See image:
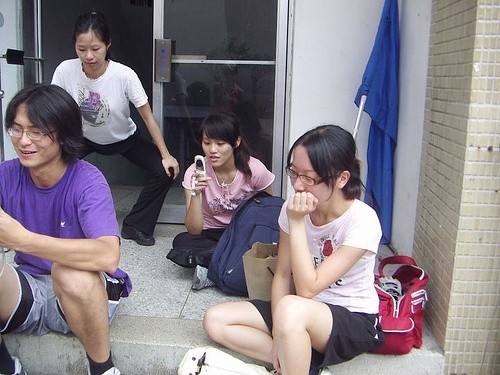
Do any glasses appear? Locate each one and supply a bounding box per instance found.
[285,162,337,186]
[8,126,57,140]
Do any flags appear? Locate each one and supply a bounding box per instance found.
[355,0,400,244]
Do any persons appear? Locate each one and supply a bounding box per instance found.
[166,110,276,290]
[203,126,384,375]
[0,84,132,375]
[51,12,179,245]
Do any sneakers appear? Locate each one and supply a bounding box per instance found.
[191,265,215,290]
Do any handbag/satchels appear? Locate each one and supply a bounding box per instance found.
[368,254,429,356]
[178,346,271,375]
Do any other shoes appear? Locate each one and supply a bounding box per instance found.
[87,361,121,375]
[10,356,27,375]
[120,223,155,246]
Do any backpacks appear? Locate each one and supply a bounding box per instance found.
[207,190,286,298]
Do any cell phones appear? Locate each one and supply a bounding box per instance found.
[194,155,206,178]
